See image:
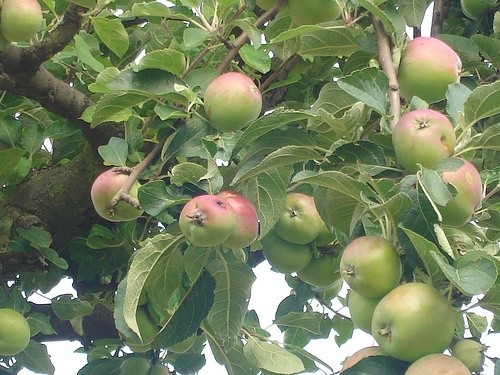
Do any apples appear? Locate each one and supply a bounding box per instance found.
[180,35,488,375]
[0,307,30,357]
[91,169,144,222]
[0,0,42,41]
